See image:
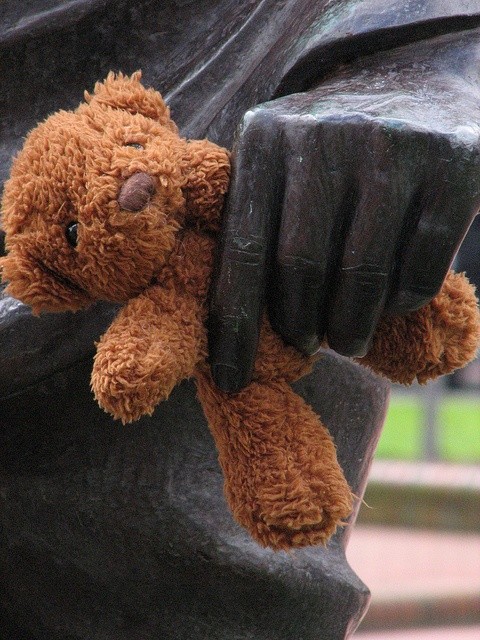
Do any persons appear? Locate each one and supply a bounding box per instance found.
[0,1,480,640]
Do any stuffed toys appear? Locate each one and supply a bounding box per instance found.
[1,70,479,550]
